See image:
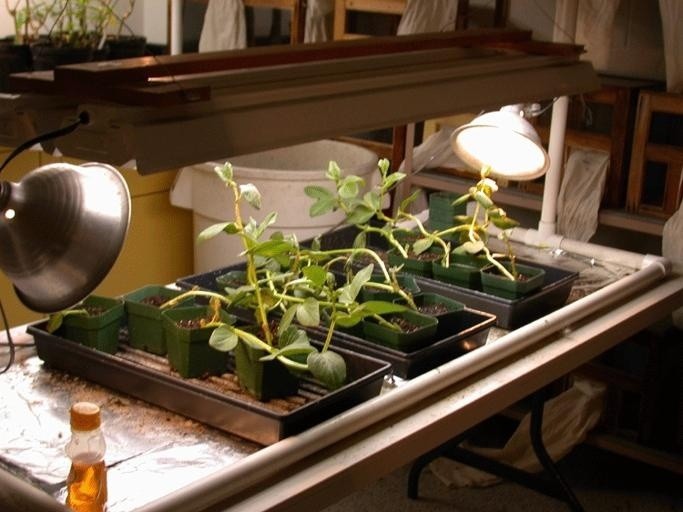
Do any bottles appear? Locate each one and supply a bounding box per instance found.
[63,400,110,512]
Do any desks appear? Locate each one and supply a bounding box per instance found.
[1,234,683,511]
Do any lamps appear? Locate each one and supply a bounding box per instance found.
[449,102,551,183]
[1,160,132,314]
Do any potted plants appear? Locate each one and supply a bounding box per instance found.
[0,1,146,66]
[48,297,125,354]
[166,158,579,394]
[125,276,232,351]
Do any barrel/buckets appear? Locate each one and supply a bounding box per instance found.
[170,139,390,275]
